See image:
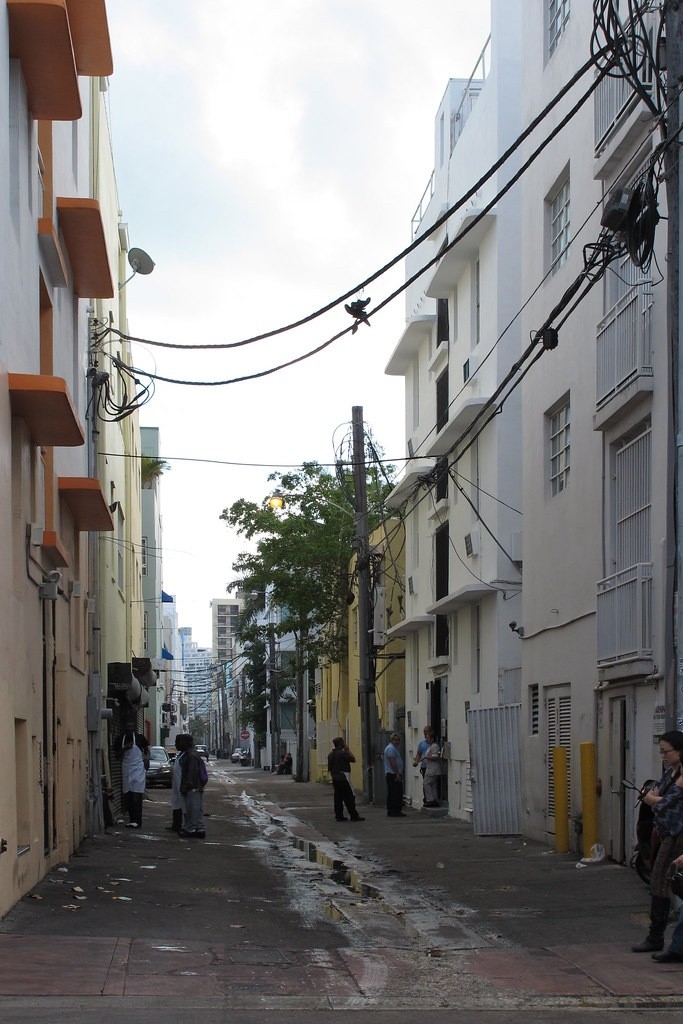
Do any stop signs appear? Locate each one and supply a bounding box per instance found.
[241,730,250,739]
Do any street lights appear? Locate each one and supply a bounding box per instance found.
[269,406,377,803]
[251,589,301,784]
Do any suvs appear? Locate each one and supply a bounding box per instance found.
[231,747,242,762]
[195,744,210,759]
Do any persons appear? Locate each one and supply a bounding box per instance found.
[631,731,683,952]
[328,737,365,821]
[113,720,150,828]
[651,826,683,962]
[384,734,407,817]
[165,734,206,839]
[413,726,433,804]
[272,753,292,775]
[423,732,442,807]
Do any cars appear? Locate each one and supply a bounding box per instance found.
[145,745,176,789]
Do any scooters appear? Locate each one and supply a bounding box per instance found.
[621,779,683,901]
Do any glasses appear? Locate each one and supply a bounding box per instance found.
[659,748,675,754]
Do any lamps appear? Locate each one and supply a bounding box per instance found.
[39,570,62,600]
[106,698,120,706]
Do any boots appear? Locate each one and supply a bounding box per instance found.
[631,896,670,952]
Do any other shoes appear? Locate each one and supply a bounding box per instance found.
[126,822,141,827]
[388,811,406,817]
[197,831,206,839]
[351,817,366,821]
[337,818,349,821]
[652,950,682,962]
[179,831,198,837]
[272,772,278,774]
[424,801,438,807]
